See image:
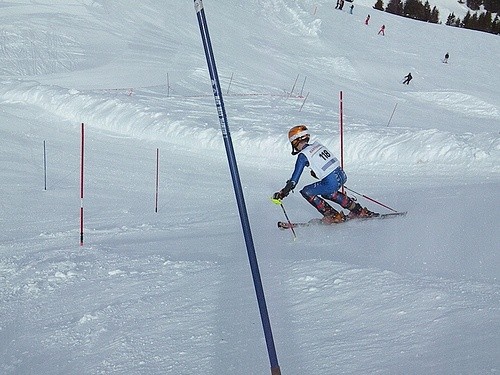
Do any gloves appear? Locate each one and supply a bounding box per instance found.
[273,192,287,200]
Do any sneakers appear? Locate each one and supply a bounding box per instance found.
[347,203,368,218]
[320,211,345,225]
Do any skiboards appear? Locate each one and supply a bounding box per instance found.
[277,211,408,231]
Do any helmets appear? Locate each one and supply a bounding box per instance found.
[288,125,310,143]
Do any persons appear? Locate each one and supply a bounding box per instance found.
[335,0,354,14]
[443,52,449,63]
[365,14,370,25]
[403,72,412,85]
[377,25,385,36]
[272,125,374,224]
